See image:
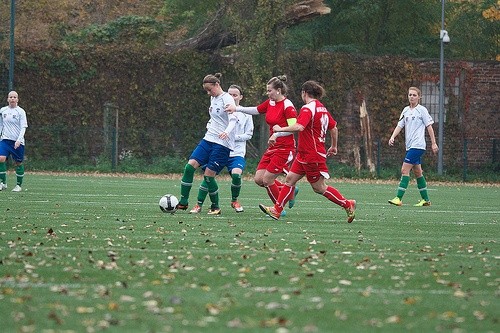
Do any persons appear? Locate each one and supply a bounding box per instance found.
[189,84,254,214]
[0,91,28,191]
[223,74,299,216]
[258,81,357,223]
[176,73,239,214]
[388,86,439,207]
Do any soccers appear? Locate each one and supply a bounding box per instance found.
[158,194,179,213]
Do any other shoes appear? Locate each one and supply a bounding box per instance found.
[12,185,22,191]
[0,182,7,190]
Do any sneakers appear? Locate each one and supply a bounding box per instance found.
[207,208,221,215]
[190,204,201,213]
[388,196,402,206]
[413,199,431,207]
[259,204,281,220]
[230,201,244,212]
[178,201,188,210]
[345,199,356,223]
[289,187,299,208]
[280,209,286,216]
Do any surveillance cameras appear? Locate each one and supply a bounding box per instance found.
[442,36,450,44]
